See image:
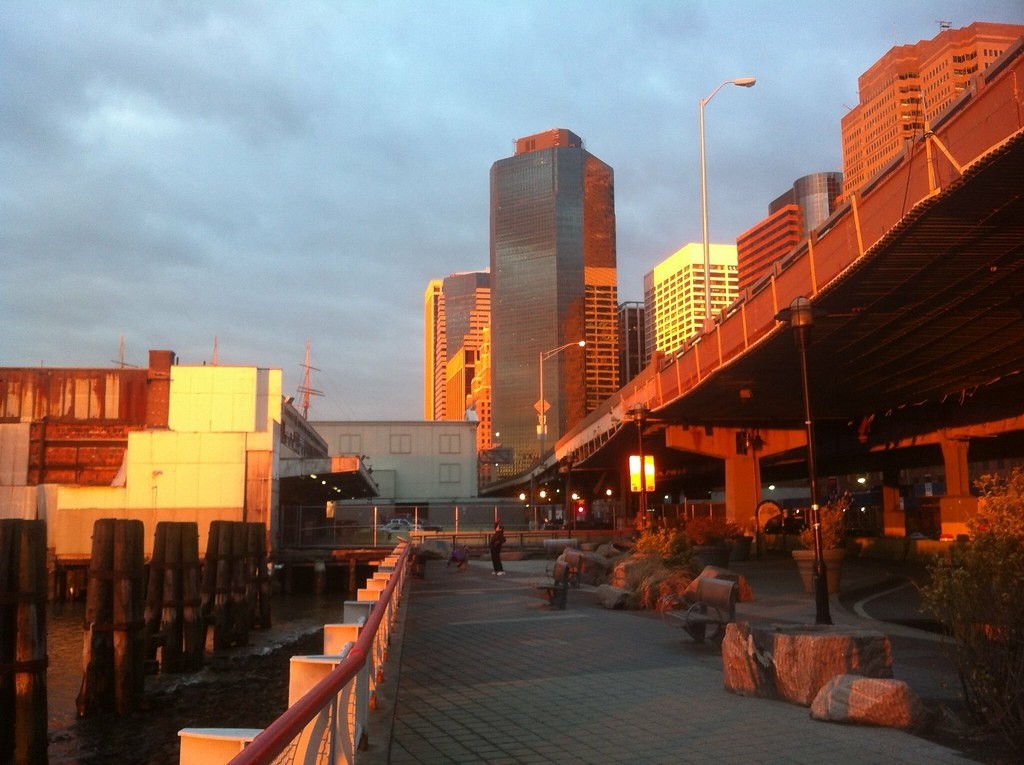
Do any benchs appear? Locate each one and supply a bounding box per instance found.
[664,577,738,647]
[537,562,571,609]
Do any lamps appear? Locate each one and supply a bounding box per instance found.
[744,430,754,448]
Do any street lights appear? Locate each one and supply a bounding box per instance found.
[626,402,652,535]
[775,296,836,627]
[699,78,757,319]
[540,341,586,465]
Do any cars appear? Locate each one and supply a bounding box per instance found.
[375,517,443,532]
[907,531,938,540]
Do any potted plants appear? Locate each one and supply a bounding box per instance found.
[791,492,856,596]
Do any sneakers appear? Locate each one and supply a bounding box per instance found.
[497,572,506,575]
[492,571,497,574]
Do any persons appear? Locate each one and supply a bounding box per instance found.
[490,518,506,576]
